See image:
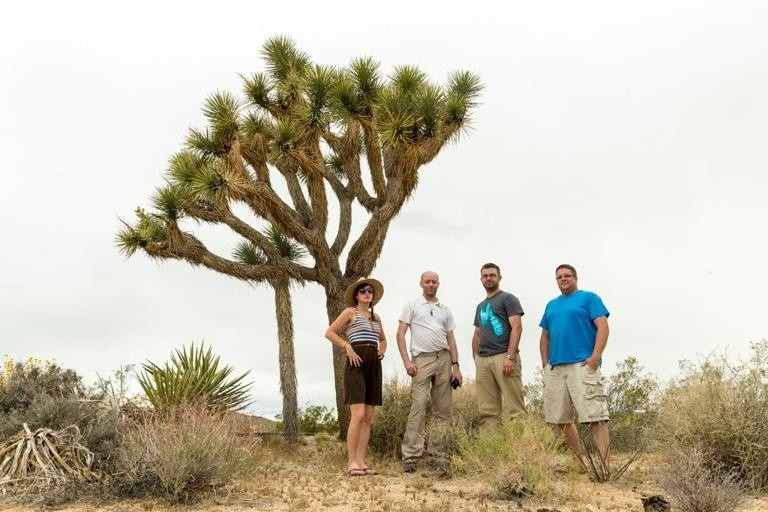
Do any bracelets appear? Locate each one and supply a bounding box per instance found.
[343,342,348,348]
[452,362,458,365]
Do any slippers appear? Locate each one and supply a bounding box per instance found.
[349,468,377,476]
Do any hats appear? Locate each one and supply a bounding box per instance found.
[344,277,384,307]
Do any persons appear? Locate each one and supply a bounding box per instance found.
[325,276,387,476]
[538,263,613,480]
[395,271,463,472]
[471,262,524,439]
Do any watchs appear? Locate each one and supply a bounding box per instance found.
[504,354,515,361]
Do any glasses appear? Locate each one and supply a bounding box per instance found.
[359,288,374,293]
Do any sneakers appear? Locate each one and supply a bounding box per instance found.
[403,463,416,472]
